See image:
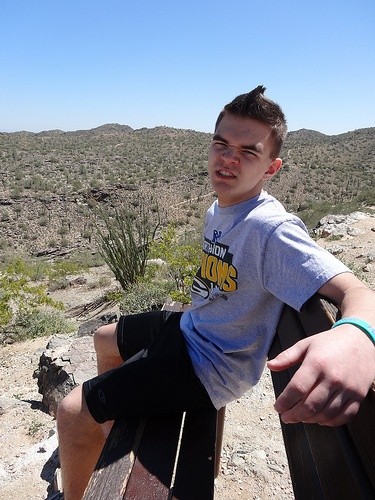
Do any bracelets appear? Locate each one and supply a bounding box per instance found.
[331,317,375,345]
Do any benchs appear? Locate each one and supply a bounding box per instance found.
[81,299,375,499]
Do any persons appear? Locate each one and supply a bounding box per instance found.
[57,85,375,500]
